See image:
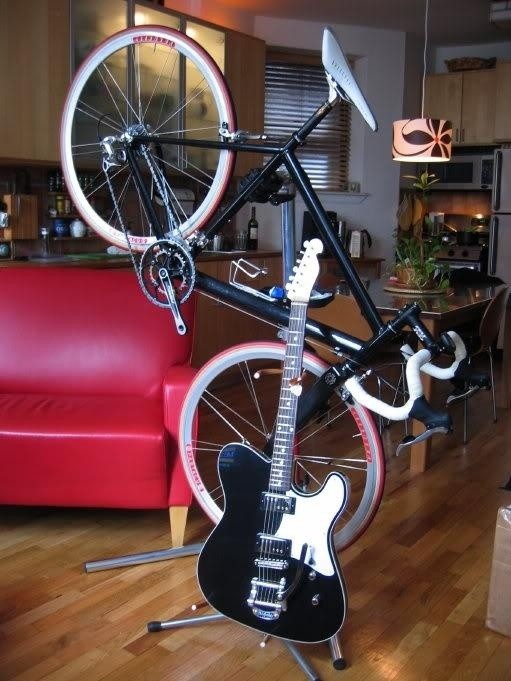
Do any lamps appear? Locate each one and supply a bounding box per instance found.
[392,0,452,163]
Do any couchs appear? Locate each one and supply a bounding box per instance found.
[0,267,198,548]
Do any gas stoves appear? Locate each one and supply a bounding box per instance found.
[435,235,486,251]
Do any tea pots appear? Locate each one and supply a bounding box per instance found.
[347,229,371,258]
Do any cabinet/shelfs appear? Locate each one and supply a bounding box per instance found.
[0,0,265,177]
[192,254,282,380]
[425,61,511,147]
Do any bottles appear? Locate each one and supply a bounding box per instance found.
[46,173,93,190]
[56,196,65,214]
[51,238,63,257]
[246,206,258,251]
[40,223,50,258]
[65,195,71,213]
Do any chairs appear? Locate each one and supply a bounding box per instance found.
[386,285,511,444]
[305,295,408,439]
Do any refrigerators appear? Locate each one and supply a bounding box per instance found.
[487,149,511,349]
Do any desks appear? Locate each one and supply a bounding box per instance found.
[365,275,496,473]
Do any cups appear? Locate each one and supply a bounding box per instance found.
[233,230,246,250]
[54,219,66,237]
[348,177,360,192]
[0,212,9,229]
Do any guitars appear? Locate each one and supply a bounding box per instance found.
[195,238,348,644]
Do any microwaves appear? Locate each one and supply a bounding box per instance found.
[420,155,494,191]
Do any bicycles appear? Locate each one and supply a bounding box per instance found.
[59,26,486,560]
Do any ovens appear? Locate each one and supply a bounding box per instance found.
[435,260,480,271]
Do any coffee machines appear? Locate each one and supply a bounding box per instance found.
[302,211,345,256]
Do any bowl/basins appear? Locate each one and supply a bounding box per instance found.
[338,278,369,295]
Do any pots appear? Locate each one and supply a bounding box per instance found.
[456,231,479,246]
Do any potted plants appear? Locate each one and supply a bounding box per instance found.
[383,170,454,294]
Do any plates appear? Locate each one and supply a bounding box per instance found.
[205,250,247,256]
